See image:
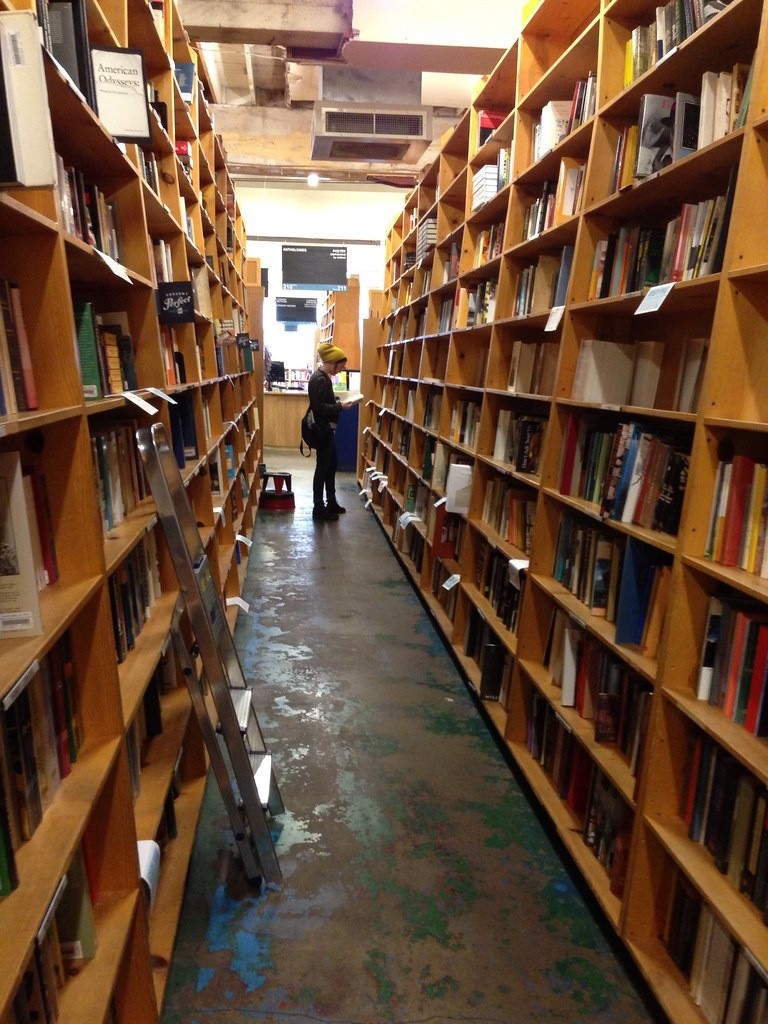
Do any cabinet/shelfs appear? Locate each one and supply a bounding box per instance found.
[314,290,361,373]
[355,0,767,1024]
[0,0,267,1024]
[271,368,313,391]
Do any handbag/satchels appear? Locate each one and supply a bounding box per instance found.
[301,409,328,450]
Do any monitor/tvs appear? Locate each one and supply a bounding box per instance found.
[267,361,285,382]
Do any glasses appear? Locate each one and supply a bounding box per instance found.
[337,364,347,370]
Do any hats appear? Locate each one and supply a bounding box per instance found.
[316,343,346,363]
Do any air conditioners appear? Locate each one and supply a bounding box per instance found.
[310,101,433,164]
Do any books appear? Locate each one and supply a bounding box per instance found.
[0,0,766,1024]
[339,388,364,409]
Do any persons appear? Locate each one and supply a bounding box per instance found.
[308,341,353,520]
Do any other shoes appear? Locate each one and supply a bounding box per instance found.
[326,501,346,514]
[312,507,339,521]
